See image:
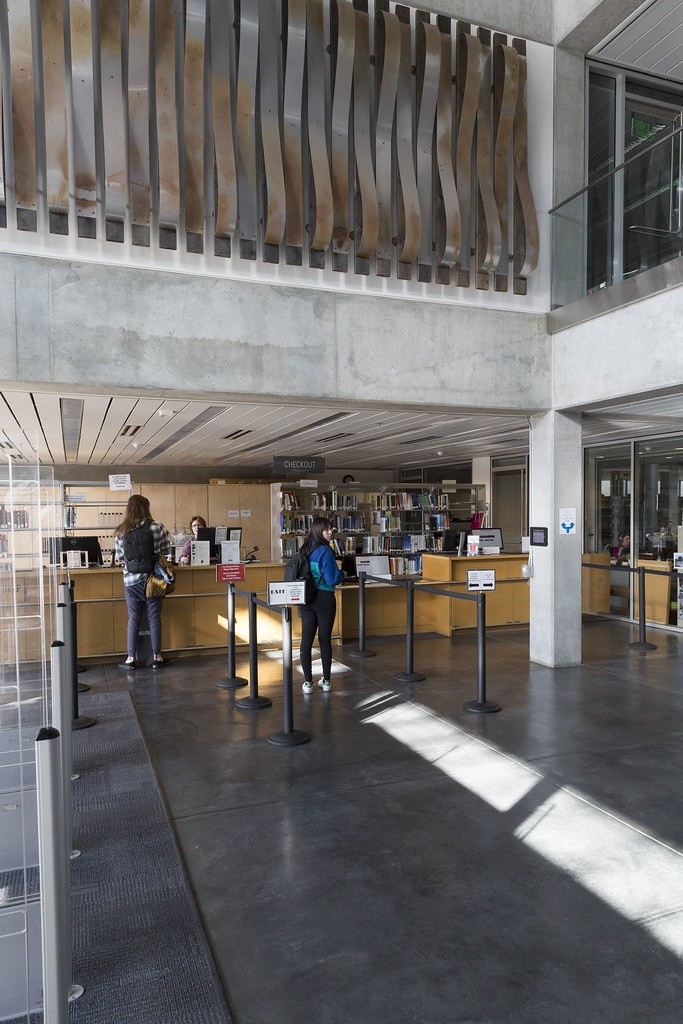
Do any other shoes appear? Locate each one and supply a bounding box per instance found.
[152,658,171,668]
[119,661,135,670]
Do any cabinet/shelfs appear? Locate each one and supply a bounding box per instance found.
[633,559,677,626]
[580,552,630,614]
[269,480,491,577]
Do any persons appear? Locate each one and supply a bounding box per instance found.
[612,533,630,556]
[113,495,170,670]
[284,517,347,693]
[180,516,206,564]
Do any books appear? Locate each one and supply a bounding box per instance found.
[282,491,486,576]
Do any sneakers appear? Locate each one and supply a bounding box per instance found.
[318,679,331,692]
[302,682,313,693]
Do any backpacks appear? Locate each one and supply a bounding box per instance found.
[282,541,328,604]
[125,520,155,574]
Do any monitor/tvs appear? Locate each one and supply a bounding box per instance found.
[342,554,373,575]
[442,530,471,550]
[198,528,243,557]
[50,537,100,562]
[530,527,548,546]
[354,554,390,580]
[470,528,504,549]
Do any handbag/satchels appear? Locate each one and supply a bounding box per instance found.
[145,553,177,600]
